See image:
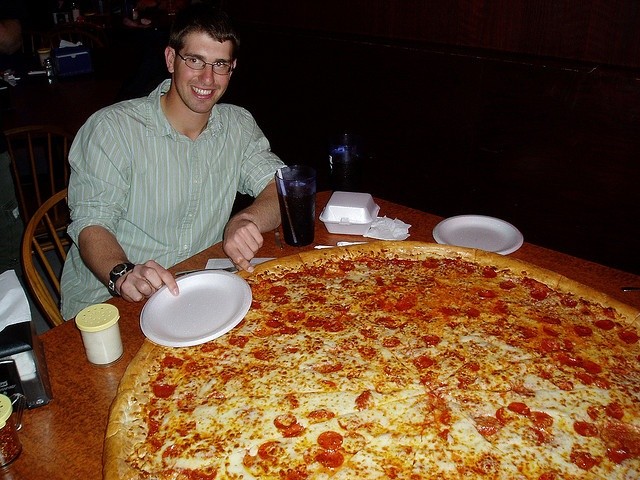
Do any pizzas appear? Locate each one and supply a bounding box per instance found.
[103,239,640,479]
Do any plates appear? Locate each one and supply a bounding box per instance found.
[431,214,525,256]
[139,268,253,349]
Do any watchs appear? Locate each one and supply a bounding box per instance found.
[108,262,136,298]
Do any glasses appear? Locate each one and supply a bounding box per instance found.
[174,53,236,74]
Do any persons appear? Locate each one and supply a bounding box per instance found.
[59,15,295,323]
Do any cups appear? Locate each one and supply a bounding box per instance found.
[274,163,317,248]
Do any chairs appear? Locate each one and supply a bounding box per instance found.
[1,125,73,256]
[42,29,103,49]
[21,184,71,328]
[19,29,54,54]
